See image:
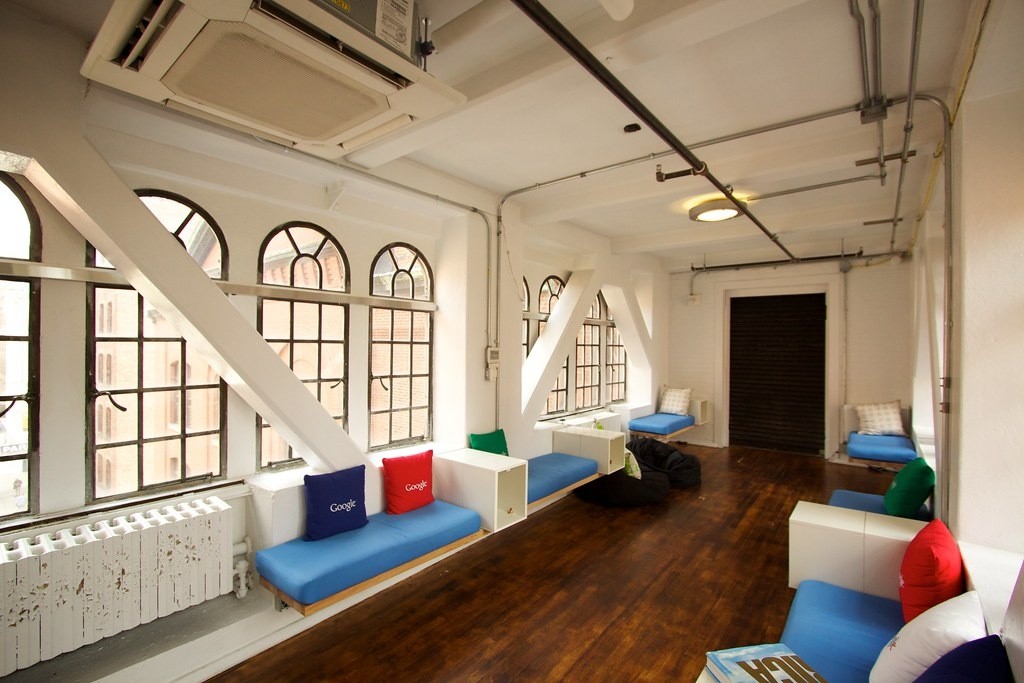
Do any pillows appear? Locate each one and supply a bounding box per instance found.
[303,464,370,541]
[382,449,435,514]
[898,518,964,623]
[469,429,508,456]
[657,384,693,416]
[883,457,936,518]
[855,398,908,436]
[912,634,1016,683]
[869,591,987,683]
[622,448,642,480]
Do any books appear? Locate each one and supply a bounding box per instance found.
[694,643,829,683]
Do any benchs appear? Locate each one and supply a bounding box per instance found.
[528,452,601,510]
[254,497,485,617]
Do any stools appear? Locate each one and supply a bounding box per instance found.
[779,578,906,683]
[846,431,917,470]
[828,489,932,521]
[628,412,695,440]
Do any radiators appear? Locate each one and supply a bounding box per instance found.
[0,494,252,679]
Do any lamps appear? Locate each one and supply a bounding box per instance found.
[688,199,747,222]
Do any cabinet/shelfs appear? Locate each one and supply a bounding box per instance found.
[553,426,625,475]
[689,398,709,425]
[788,499,932,602]
[434,448,529,532]
